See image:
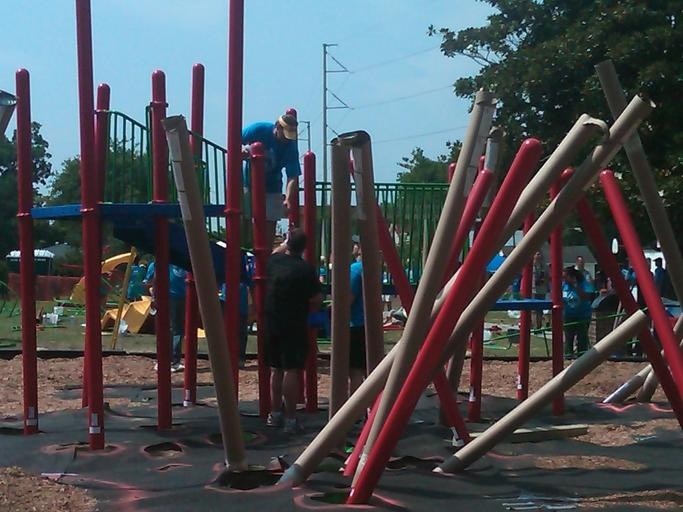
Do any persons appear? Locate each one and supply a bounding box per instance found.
[242,116,302,253]
[401,253,420,288]
[480,227,682,360]
[138,227,396,427]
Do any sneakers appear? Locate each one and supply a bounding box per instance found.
[153,362,185,373]
[266,412,285,427]
[283,419,305,434]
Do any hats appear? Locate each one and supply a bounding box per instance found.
[287,228,306,251]
[278,115,298,141]
